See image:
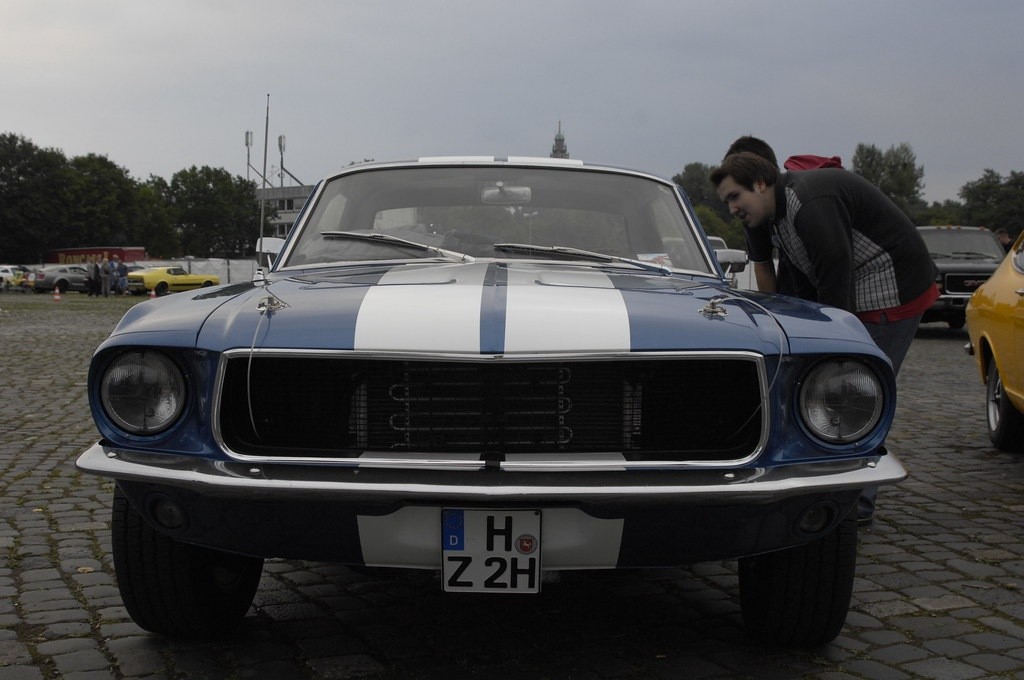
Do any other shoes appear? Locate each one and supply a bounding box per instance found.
[856,512,873,525]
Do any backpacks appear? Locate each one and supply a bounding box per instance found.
[784,154,844,171]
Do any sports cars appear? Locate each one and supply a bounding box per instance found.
[127,266,220,296]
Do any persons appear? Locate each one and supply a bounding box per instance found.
[87,254,129,298]
[710,136,943,525]
[995,227,1016,254]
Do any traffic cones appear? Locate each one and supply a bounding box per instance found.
[54,286,62,302]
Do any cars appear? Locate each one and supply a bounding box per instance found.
[662,237,737,289]
[912,224,1008,331]
[81,156,910,653]
[30,265,89,295]
[0,265,35,291]
[964,227,1024,452]
